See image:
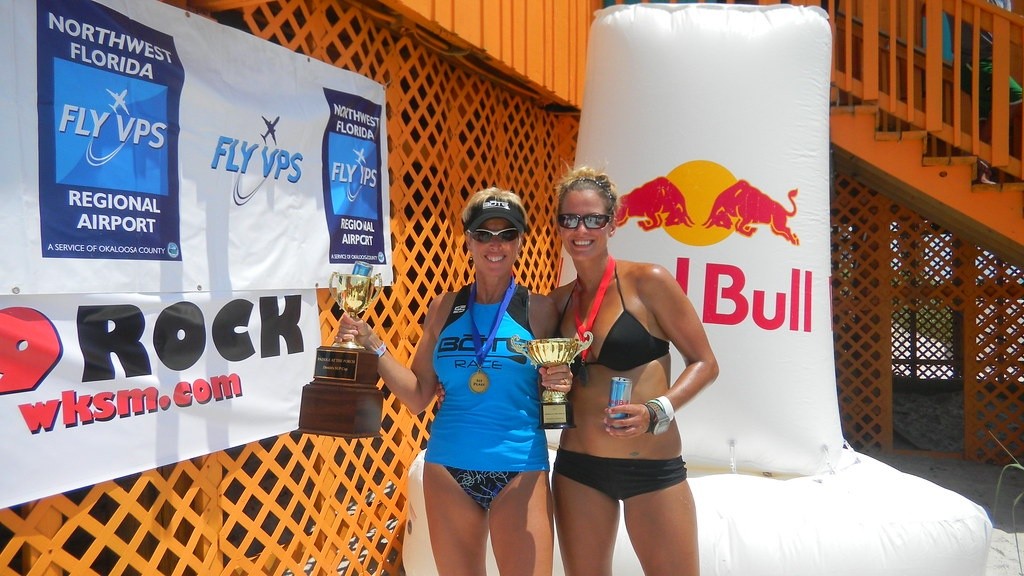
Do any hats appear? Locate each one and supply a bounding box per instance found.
[463,196,529,235]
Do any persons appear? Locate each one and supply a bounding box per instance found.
[435,170,717,575]
[334,187,574,575]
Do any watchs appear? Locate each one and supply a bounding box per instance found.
[647,402,670,436]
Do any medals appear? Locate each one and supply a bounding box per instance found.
[470,366,491,395]
[578,362,591,386]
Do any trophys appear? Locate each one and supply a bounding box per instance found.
[511,330,594,429]
[298,262,384,438]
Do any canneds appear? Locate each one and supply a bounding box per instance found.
[607,376,632,429]
[352,260,374,277]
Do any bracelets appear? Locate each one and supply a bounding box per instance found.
[644,396,674,433]
[372,343,387,360]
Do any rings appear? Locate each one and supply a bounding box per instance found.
[564,378,570,385]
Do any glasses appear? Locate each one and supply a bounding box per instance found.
[466,228,520,243]
[558,212,613,229]
